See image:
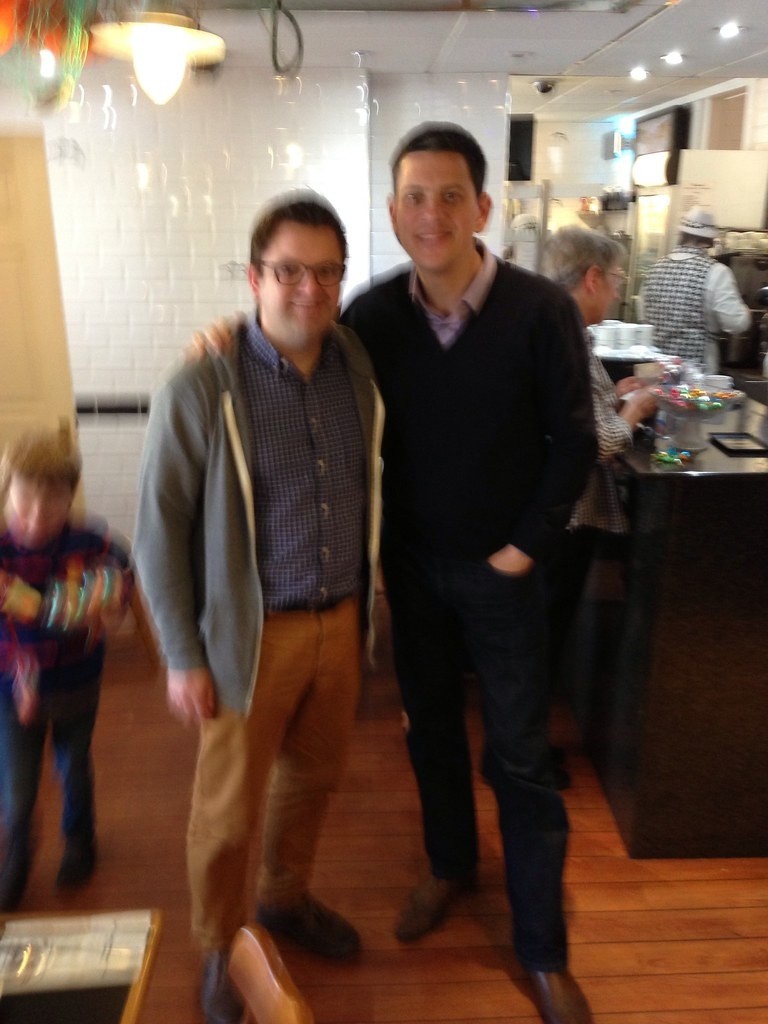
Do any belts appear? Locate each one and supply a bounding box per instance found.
[278,594,348,612]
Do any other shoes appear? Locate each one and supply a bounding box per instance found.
[202,948,242,1024]
[0,842,28,909]
[56,840,94,890]
[257,894,359,958]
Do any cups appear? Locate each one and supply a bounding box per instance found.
[589,319,655,348]
[708,374,734,390]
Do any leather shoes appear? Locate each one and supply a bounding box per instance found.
[526,968,591,1024]
[395,873,462,942]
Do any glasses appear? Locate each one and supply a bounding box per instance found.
[603,270,631,287]
[259,261,345,287]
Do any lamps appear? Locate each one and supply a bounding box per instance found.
[97,0,227,103]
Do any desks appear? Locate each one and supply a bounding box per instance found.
[0,907,163,1024]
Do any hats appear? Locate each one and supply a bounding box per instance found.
[678,206,719,239]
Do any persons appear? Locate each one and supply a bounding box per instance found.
[181,122,600,1024]
[548,227,659,786]
[131,190,386,1024]
[643,205,751,375]
[0,431,137,910]
